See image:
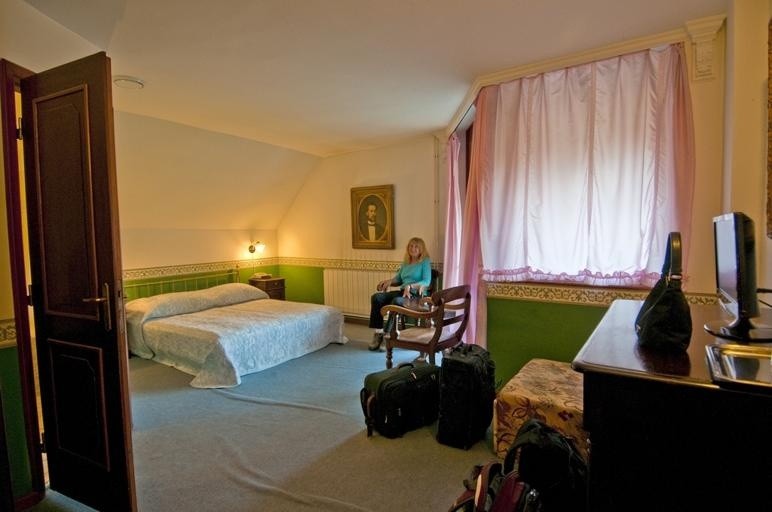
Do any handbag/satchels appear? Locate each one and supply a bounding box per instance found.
[634,231,692,355]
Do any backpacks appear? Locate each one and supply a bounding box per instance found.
[448,417,587,512]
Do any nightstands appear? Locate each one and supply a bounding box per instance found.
[248,277,285,301]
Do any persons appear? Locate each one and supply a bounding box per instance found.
[360,203,384,239]
[366,237,433,349]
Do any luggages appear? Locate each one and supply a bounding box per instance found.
[436,341,496,450]
[361,355,440,438]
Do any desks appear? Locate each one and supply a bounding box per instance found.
[573,299,771,512]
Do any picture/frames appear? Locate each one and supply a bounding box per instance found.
[349,184,395,251]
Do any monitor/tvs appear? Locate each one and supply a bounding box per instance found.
[704,211,772,345]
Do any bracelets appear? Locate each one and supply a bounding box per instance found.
[409,284,412,289]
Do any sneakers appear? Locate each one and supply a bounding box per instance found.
[368,331,398,352]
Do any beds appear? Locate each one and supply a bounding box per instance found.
[122,265,351,395]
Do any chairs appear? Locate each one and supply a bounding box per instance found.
[380,284,473,368]
